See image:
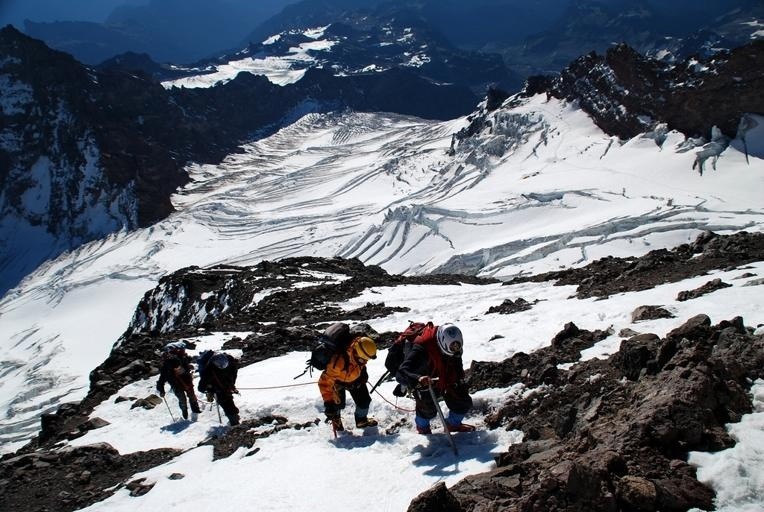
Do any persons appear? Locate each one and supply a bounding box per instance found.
[395,322,476,436]
[197,352,240,427]
[156,356,200,421]
[317,337,377,429]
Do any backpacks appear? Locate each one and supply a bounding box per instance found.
[306,322,353,372]
[385,320,434,377]
[164,343,185,364]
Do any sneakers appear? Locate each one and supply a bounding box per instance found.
[182,410,188,419]
[357,418,378,428]
[444,423,475,432]
[332,420,344,431]
[192,407,202,412]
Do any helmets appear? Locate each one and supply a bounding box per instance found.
[214,354,228,369]
[436,323,463,357]
[355,337,377,360]
